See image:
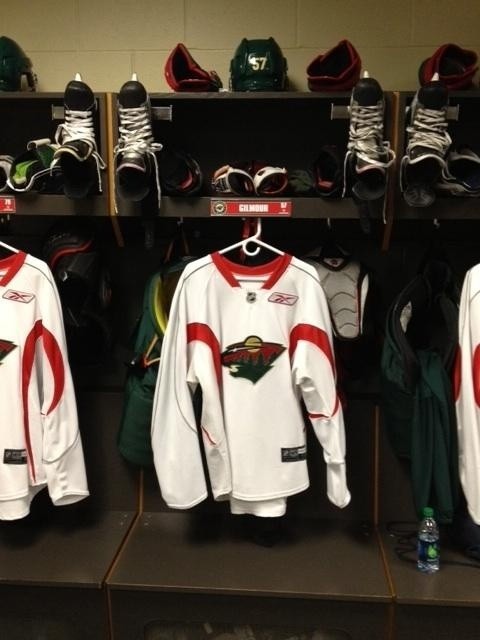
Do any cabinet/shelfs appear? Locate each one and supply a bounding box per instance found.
[2,88,480,220]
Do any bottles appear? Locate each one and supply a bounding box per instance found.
[417,509,440,576]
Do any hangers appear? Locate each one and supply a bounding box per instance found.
[183,216,319,279]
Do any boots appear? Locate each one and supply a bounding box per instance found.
[347,78,390,201]
[399,81,452,208]
[114,81,164,202]
[49,80,108,199]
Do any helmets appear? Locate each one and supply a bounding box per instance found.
[229,36,288,92]
[0,35,38,92]
